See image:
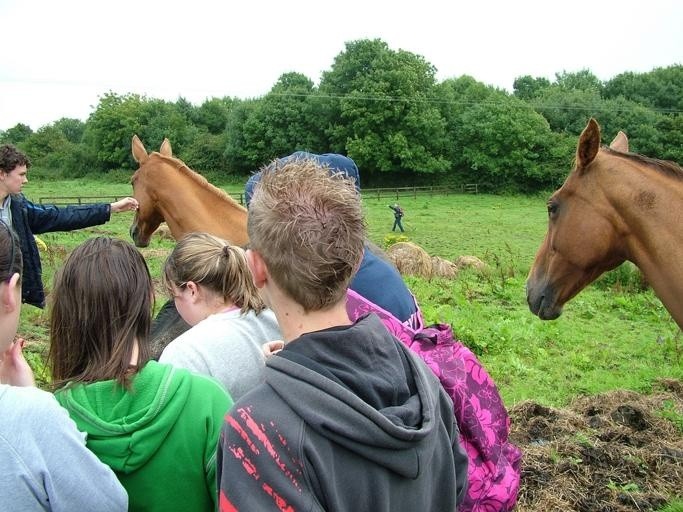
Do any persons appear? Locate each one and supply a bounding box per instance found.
[214,160,467,511]
[154,228,287,400]
[0,218,129,512]
[47,237,235,511]
[0,145,143,315]
[246,152,423,338]
[387,203,404,232]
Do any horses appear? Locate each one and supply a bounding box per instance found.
[524,117,683,333]
[129,133,250,249]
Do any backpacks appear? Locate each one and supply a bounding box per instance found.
[345,288,522,512]
